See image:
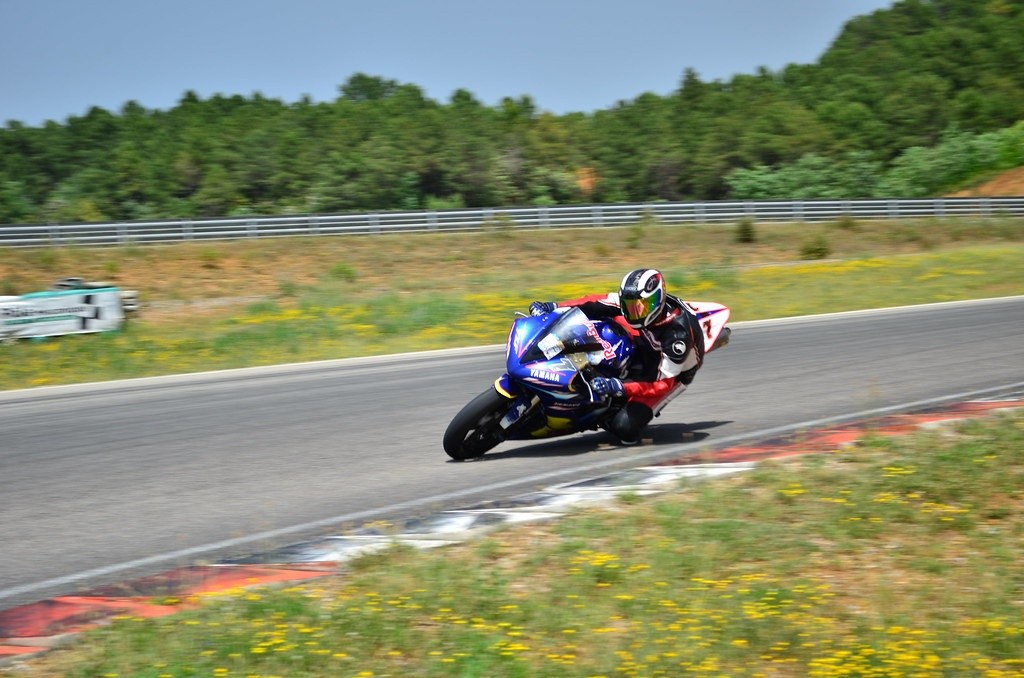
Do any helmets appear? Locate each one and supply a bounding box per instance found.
[618,267,667,329]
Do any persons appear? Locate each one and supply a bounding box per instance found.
[529,268,705,445]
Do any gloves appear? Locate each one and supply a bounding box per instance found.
[589,376,624,394]
[529,300,558,317]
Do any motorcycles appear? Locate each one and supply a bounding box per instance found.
[442,301,732,461]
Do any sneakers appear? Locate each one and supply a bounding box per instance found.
[621,425,643,444]
